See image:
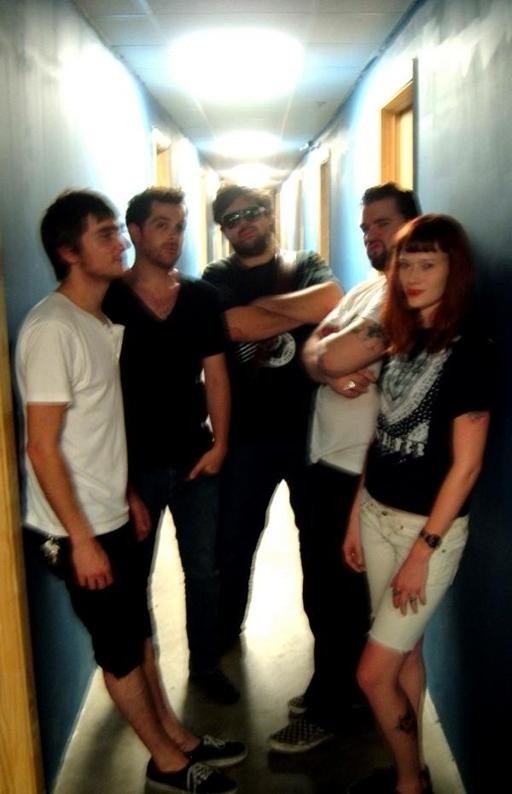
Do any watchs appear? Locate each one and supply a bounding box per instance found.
[418,527,442,549]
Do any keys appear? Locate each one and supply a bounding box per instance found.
[42,533,60,564]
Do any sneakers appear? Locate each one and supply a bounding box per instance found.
[181,734,250,766]
[144,751,239,793]
[184,660,244,707]
[268,696,343,755]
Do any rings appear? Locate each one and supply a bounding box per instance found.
[346,379,356,388]
[392,589,402,596]
[410,596,417,603]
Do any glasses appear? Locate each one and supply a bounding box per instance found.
[216,204,271,228]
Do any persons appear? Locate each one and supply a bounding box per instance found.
[10,185,249,792]
[101,188,249,708]
[264,176,425,757]
[341,209,496,792]
[202,181,348,662]
